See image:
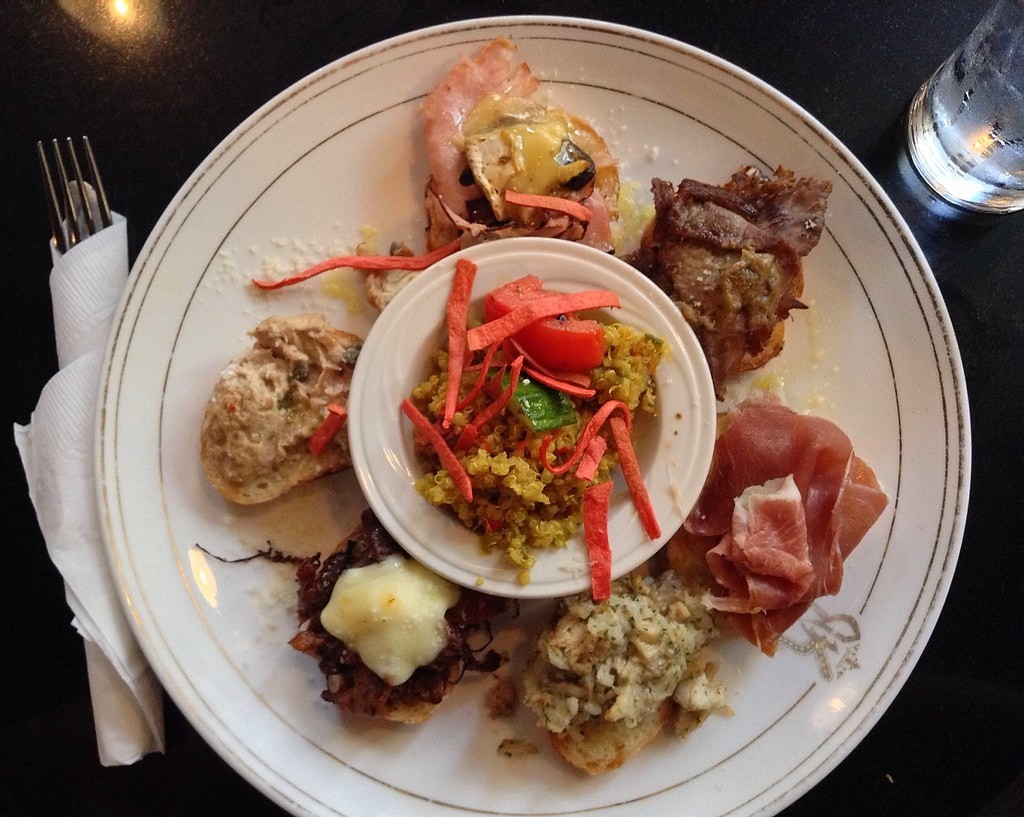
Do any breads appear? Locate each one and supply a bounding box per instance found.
[199,316,364,504]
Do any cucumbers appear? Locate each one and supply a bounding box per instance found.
[496,375,578,431]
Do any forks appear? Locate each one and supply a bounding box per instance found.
[34,136,110,255]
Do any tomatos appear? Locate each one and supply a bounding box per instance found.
[484,276,607,375]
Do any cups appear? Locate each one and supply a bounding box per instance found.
[906,0,1023,215]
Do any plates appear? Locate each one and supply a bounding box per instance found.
[95,13,969,817]
[345,236,717,600]
